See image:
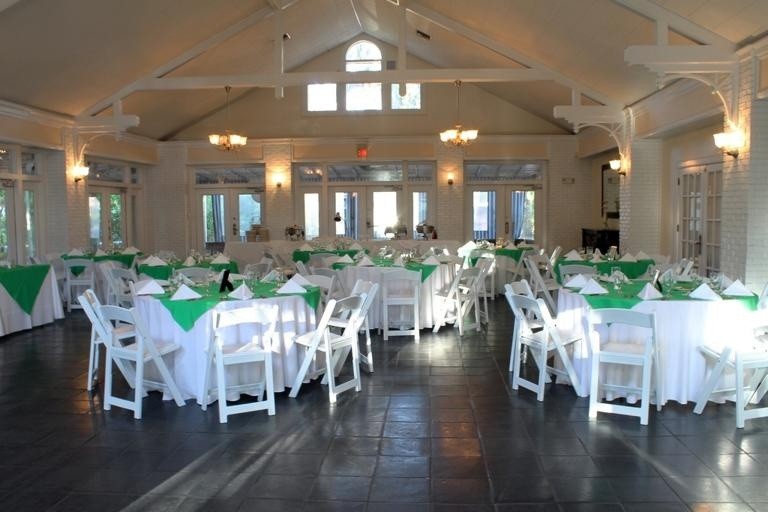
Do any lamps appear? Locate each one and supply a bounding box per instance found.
[447,172,455,185]
[712,129,747,160]
[206,84,249,152]
[69,160,91,184]
[272,173,285,188]
[438,80,480,147]
[609,159,630,178]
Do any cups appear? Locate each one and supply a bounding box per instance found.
[579,247,616,262]
[356,248,415,266]
[165,267,285,293]
[591,266,721,297]
